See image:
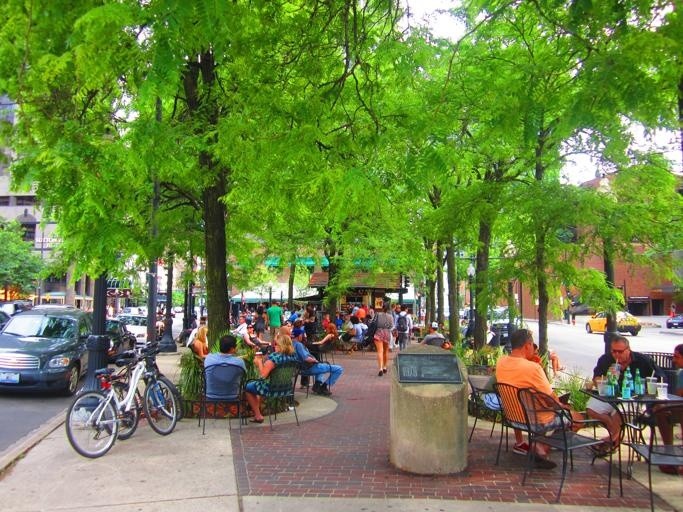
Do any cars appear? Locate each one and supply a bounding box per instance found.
[459,307,523,345]
[0,297,163,350]
[0,306,93,397]
[584,307,641,337]
[103,317,137,363]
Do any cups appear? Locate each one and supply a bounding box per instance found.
[256,352,262,359]
[597,364,668,398]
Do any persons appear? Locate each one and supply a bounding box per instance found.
[586,336,668,451]
[496,329,585,462]
[653,344,683,447]
[481,343,550,457]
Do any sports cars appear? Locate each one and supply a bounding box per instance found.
[664,314,682,329]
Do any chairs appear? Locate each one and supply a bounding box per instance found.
[464,348,682,511]
[194,314,373,435]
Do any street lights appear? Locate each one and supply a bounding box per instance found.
[266,279,273,309]
[465,263,474,338]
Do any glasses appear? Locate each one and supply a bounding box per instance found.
[609,346,629,353]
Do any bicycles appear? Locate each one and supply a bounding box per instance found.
[64,346,174,465]
[99,338,184,442]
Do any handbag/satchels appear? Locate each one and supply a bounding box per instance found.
[367,312,378,338]
[342,328,354,342]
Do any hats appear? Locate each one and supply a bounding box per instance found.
[292,327,306,338]
[431,321,438,329]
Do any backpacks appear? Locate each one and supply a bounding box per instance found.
[395,312,408,332]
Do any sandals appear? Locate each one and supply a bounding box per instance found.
[249,417,264,423]
[590,437,619,457]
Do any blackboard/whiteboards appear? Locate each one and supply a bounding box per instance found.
[397,353,463,383]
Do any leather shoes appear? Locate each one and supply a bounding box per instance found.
[379,371,383,376]
[383,369,387,373]
[312,384,321,391]
[317,387,332,395]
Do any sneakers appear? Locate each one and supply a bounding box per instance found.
[660,464,682,474]
[534,452,557,470]
[345,350,354,356]
[510,441,529,456]
